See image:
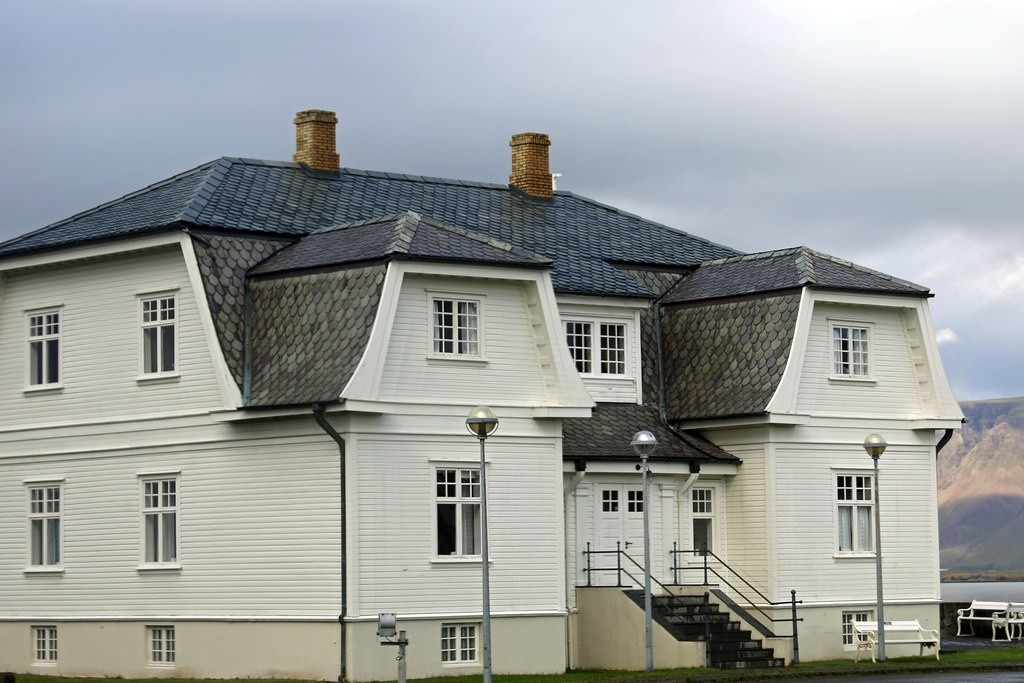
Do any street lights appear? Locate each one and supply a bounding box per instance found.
[464,404,501,683]
[630,429,660,671]
[863,432,889,660]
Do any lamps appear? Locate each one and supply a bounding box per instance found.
[376,612,397,637]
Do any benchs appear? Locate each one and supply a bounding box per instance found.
[849,619,941,664]
[991,602,1024,642]
[957,599,1020,639]
